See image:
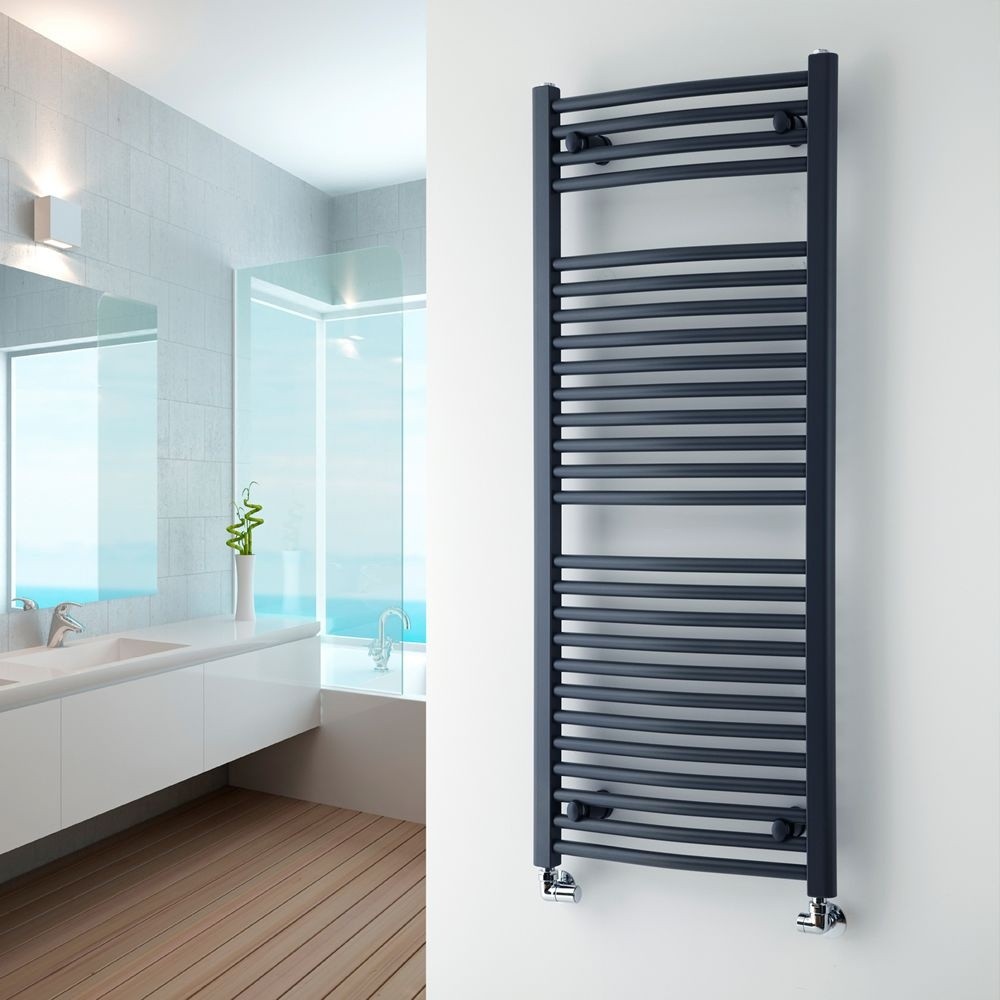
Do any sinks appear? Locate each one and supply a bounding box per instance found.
[0,635,192,678]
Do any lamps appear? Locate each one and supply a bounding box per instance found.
[34,197,82,251]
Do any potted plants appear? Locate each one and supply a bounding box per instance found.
[223,481,265,620]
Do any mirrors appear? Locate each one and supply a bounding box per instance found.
[1,264,161,612]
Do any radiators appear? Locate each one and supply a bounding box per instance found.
[525,48,847,935]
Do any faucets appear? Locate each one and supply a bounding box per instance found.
[368,606,410,673]
[45,601,84,649]
[10,596,40,612]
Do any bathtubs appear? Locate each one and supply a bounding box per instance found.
[224,633,426,827]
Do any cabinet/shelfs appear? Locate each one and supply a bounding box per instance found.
[1,631,322,856]
